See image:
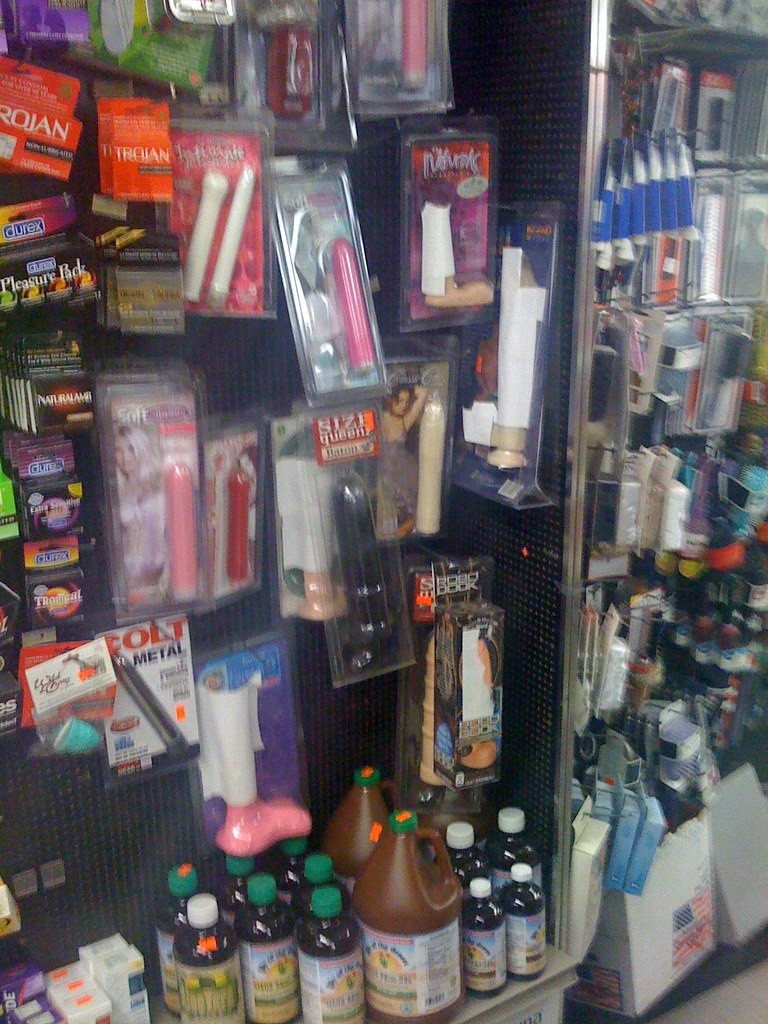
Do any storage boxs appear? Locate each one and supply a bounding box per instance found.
[567,756,768,1015]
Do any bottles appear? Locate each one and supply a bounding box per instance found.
[502,859,546,981]
[295,853,354,928]
[173,891,246,1024]
[274,832,311,904]
[354,808,468,1024]
[218,850,263,928]
[462,877,508,998]
[441,818,487,886]
[153,865,213,1017]
[484,807,544,903]
[317,766,399,903]
[295,884,366,1024]
[231,874,303,1024]
